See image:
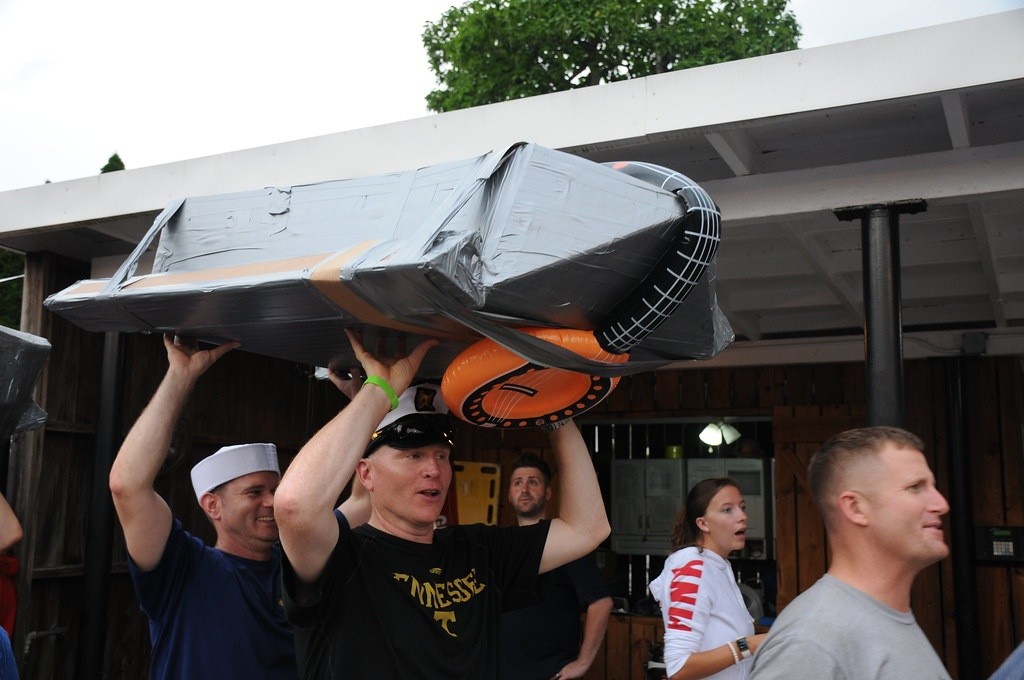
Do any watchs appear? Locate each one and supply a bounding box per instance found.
[738,637,751,658]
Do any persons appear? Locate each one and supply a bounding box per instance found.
[744,424,956,679]
[110,326,767,680]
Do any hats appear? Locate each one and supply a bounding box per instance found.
[190,442,281,504]
[361,383,458,459]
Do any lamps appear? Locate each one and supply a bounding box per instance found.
[699,419,741,453]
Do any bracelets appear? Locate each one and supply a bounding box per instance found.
[542,417,573,433]
[361,375,398,410]
[724,642,740,666]
